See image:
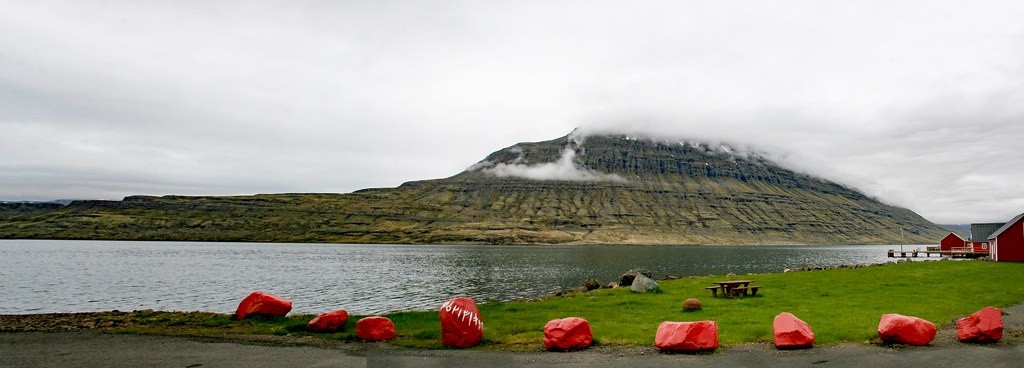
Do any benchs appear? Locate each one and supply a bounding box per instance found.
[705,284,761,298]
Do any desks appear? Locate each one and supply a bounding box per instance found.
[713,280,754,298]
